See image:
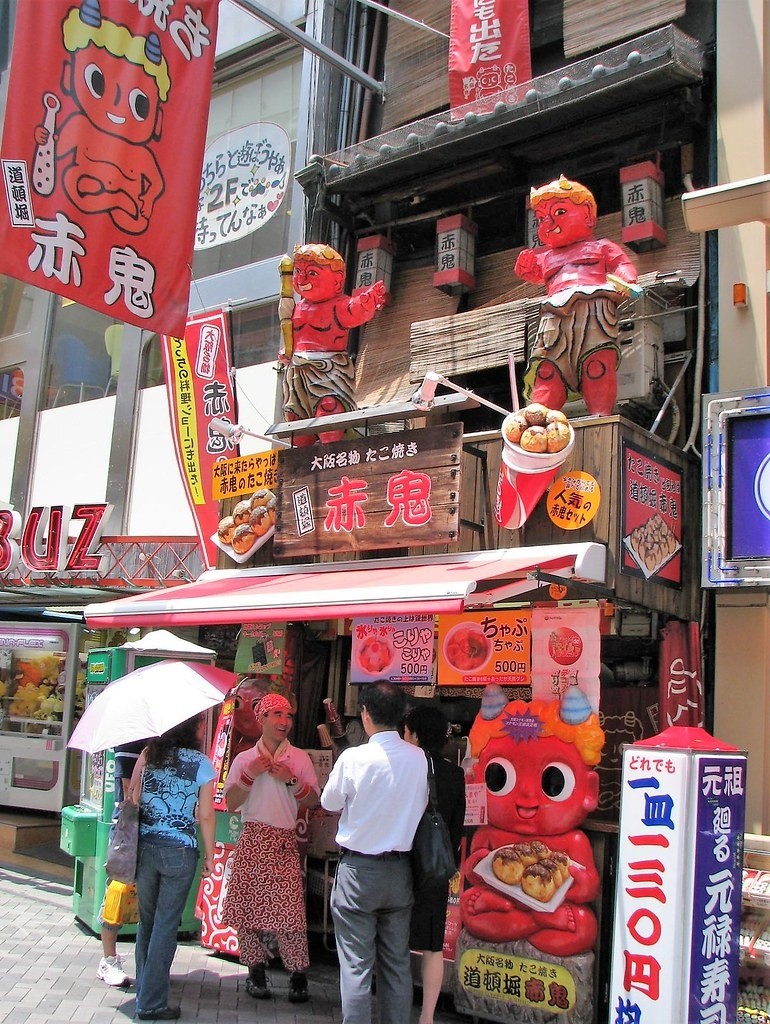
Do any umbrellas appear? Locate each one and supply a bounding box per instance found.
[66,659,237,754]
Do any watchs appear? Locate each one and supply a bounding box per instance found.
[286,775,298,786]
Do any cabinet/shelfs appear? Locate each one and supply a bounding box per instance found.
[735,833,770,1024]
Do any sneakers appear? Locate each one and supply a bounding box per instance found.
[96,954,129,987]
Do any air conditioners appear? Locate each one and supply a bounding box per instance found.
[526,289,667,414]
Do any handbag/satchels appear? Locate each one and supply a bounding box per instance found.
[106,748,150,884]
[410,746,458,886]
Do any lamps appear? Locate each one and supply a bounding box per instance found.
[139,552,192,583]
[209,416,298,449]
[732,282,750,311]
[412,370,520,416]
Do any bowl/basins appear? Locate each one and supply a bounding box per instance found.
[356,634,396,676]
[442,621,494,674]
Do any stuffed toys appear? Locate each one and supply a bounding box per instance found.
[0,655,85,733]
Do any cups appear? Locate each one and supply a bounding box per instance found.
[494,415,575,529]
[317,724,333,747]
[332,718,346,738]
[323,698,341,724]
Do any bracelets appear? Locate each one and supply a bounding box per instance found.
[237,769,256,792]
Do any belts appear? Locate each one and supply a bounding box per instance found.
[341,847,406,858]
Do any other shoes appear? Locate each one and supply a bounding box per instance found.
[138,1005,180,1020]
[246,963,273,1000]
[288,971,309,1004]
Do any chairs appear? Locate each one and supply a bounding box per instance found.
[51,335,110,408]
[104,324,163,396]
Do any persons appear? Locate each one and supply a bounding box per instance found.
[278,244,385,447]
[97,739,148,986]
[137,714,216,1019]
[513,174,637,418]
[225,694,322,1003]
[319,680,430,1023]
[401,707,465,1023]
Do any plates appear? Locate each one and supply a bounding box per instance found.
[472,844,587,913]
[209,526,275,564]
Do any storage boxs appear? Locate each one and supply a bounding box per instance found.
[103,880,139,924]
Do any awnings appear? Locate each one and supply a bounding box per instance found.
[84,543,606,628]
